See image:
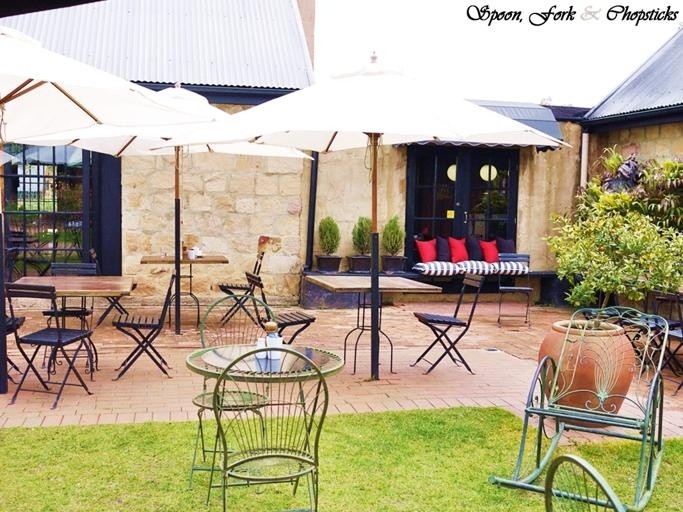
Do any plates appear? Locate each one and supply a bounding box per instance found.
[265,331,282,359]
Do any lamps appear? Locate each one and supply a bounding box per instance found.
[447,165,456,182]
[480,165,498,183]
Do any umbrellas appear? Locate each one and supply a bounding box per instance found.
[0,34,215,151]
[149,51,574,378]
[69,79,314,162]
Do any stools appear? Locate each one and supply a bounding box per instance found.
[574,273,683,398]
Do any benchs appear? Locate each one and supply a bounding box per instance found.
[413,258,531,277]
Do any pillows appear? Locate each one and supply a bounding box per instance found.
[408,234,517,261]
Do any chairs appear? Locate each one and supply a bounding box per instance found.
[487,302,670,512]
[496,252,535,326]
[541,450,629,512]
[186,293,283,488]
[242,271,317,350]
[409,272,484,377]
[203,344,332,512]
[0,208,175,410]
[218,250,266,330]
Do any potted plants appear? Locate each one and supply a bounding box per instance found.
[349,217,373,272]
[377,215,407,270]
[314,213,345,273]
[536,177,683,430]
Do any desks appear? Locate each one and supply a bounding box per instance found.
[140,255,230,337]
[182,347,348,505]
[3,275,136,376]
[304,276,444,379]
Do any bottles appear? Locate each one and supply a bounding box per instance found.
[181,241,203,260]
[256,339,266,359]
